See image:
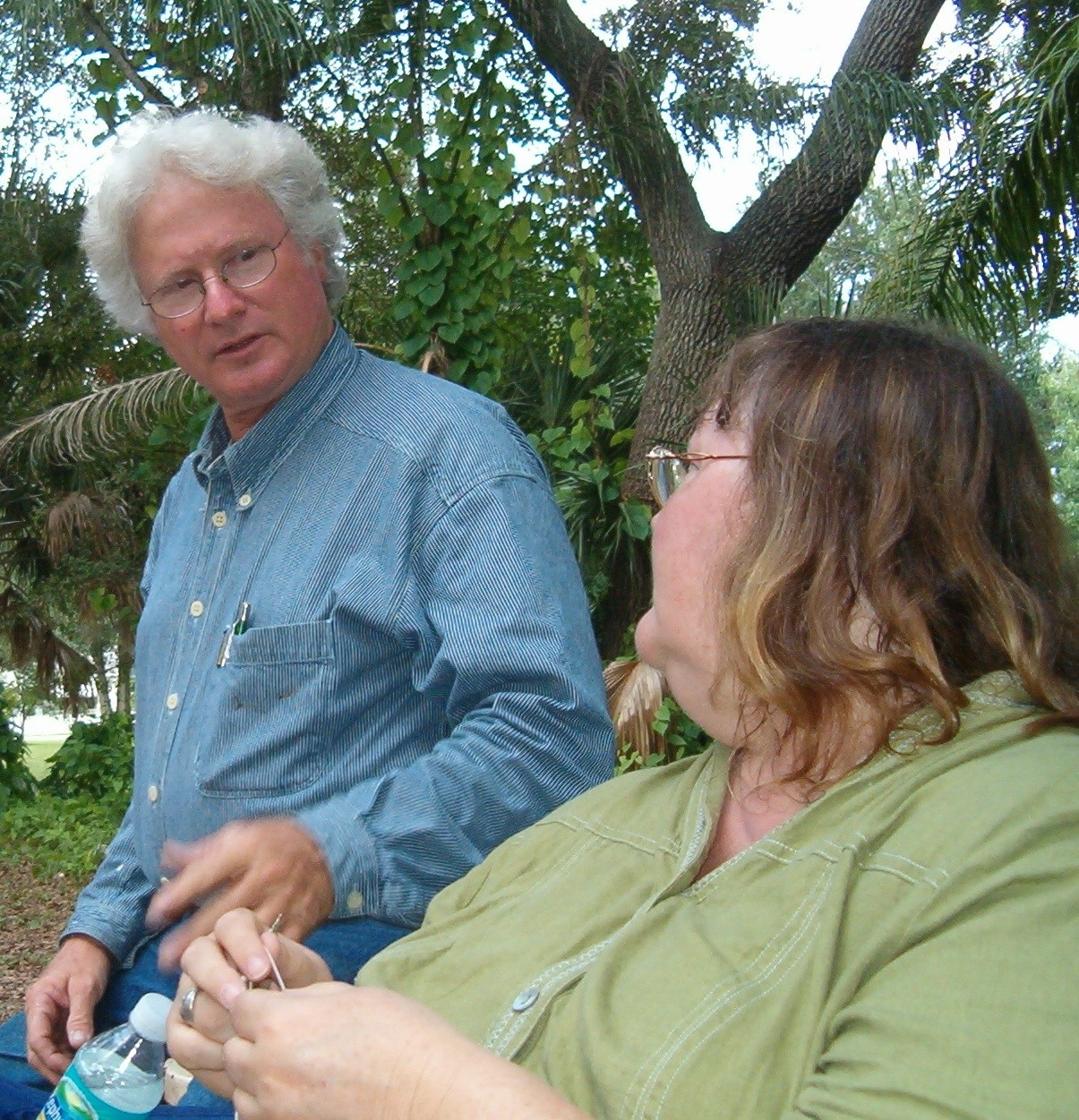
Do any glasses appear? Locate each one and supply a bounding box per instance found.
[140,228,292,319]
[645,446,754,509]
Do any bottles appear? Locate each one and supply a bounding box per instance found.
[34,992,176,1120]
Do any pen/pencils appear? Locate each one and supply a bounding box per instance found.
[216,603,251,668]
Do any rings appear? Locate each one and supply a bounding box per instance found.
[180,987,204,1026]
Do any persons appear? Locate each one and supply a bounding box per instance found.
[0,107,617,1120]
[168,316,1079,1120]
